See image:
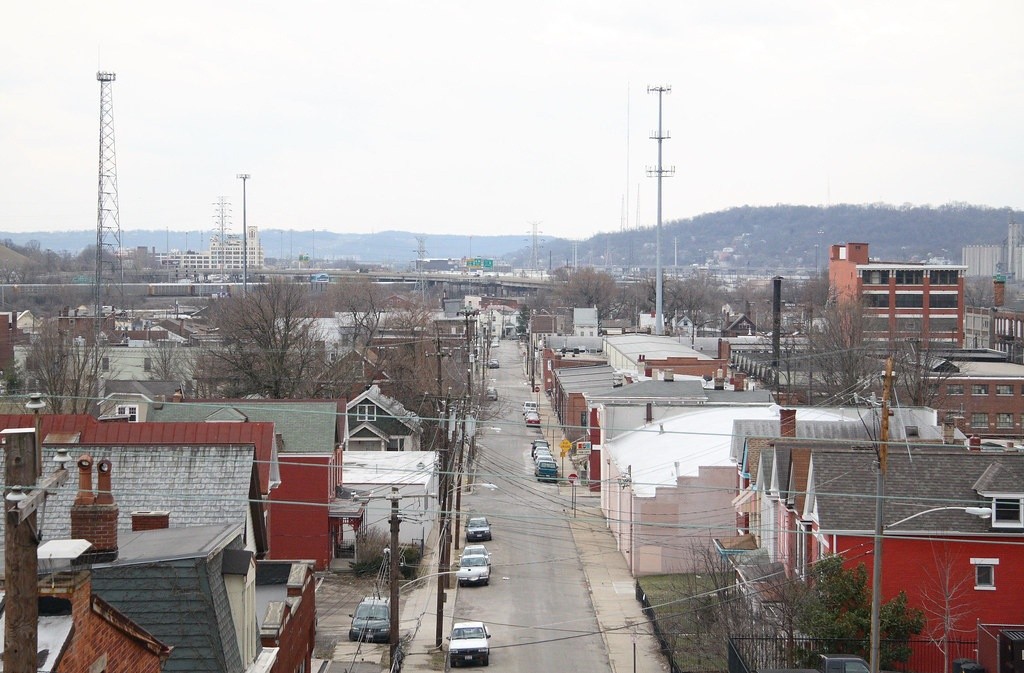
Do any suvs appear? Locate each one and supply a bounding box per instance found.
[349,594,391,644]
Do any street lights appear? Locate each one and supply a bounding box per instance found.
[443,483,498,591]
[454,427,501,550]
[388,568,475,673]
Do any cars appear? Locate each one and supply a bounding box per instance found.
[460,545,493,571]
[456,554,491,587]
[489,359,499,368]
[465,517,492,541]
[485,389,498,401]
[522,401,541,427]
[446,621,492,669]
[491,337,500,347]
[530,439,559,483]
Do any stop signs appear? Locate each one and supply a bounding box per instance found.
[535,387,540,392]
